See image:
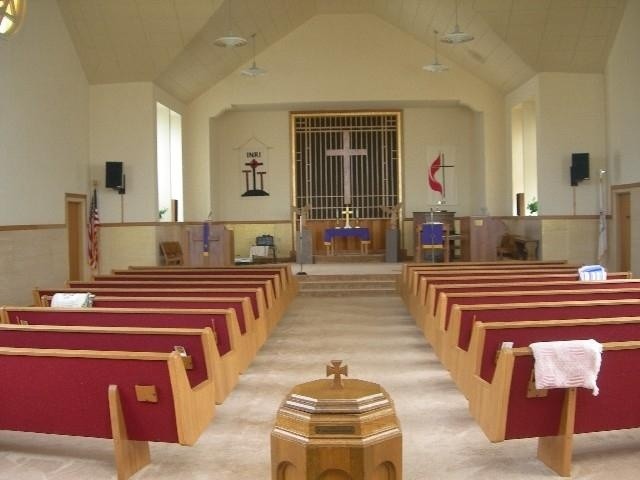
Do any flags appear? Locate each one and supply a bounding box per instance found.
[87,188,100,271]
[598,181,609,261]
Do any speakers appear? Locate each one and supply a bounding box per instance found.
[572,153,589,180]
[106,161,122,187]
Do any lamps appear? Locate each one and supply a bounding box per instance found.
[211,0,268,79]
[419,1,477,74]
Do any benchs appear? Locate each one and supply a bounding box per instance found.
[2,262,299,479]
[394,257,640,476]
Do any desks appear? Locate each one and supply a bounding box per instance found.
[325,228,369,257]
